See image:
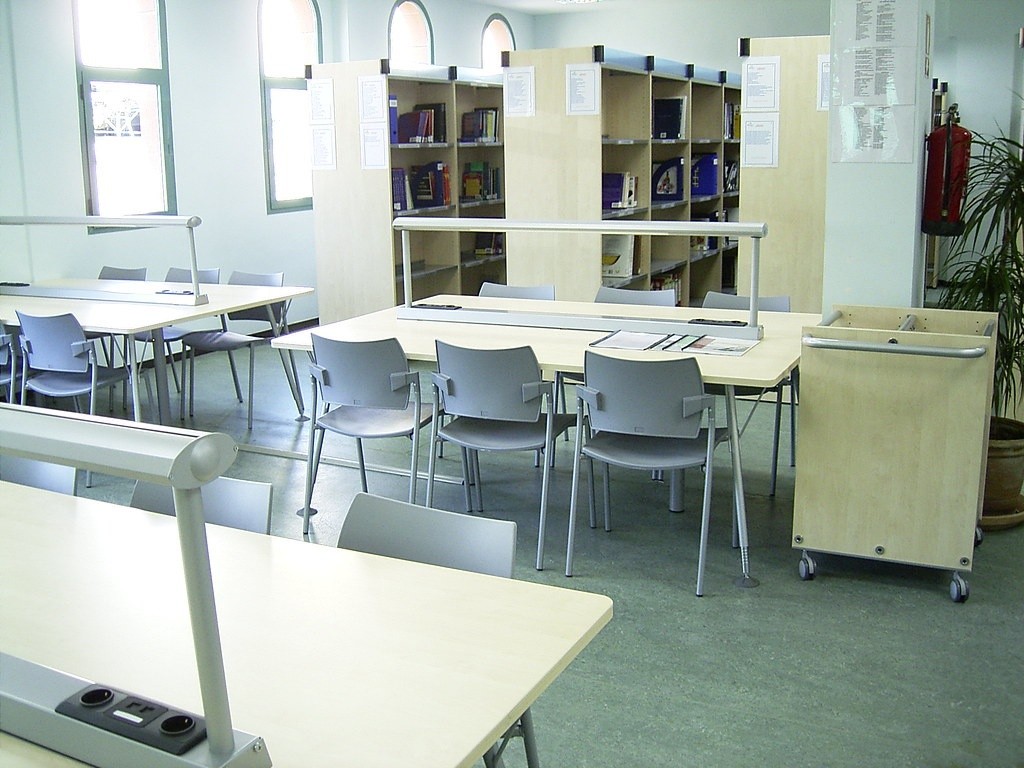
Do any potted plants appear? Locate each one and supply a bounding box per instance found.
[924,83,1024,534]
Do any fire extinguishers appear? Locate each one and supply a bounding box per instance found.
[923,100,971,236]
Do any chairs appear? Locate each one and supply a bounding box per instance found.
[129,476,273,535]
[0,454,79,497]
[0,265,800,599]
[336,492,518,767]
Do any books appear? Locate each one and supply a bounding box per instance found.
[650,268,682,307]
[602,235,643,278]
[724,102,741,142]
[398,102,447,143]
[474,232,504,255]
[690,209,738,289]
[392,160,451,211]
[457,106,499,143]
[652,96,687,140]
[395,259,426,277]
[459,160,501,204]
[723,160,739,193]
[602,171,639,209]
[652,157,684,201]
[691,152,718,195]
[589,329,761,356]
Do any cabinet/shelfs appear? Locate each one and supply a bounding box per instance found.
[500,42,740,385]
[924,80,949,290]
[736,35,831,406]
[304,57,507,328]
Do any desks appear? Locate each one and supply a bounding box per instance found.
[0,278,316,423]
[270,291,822,590]
[0,480,614,768]
